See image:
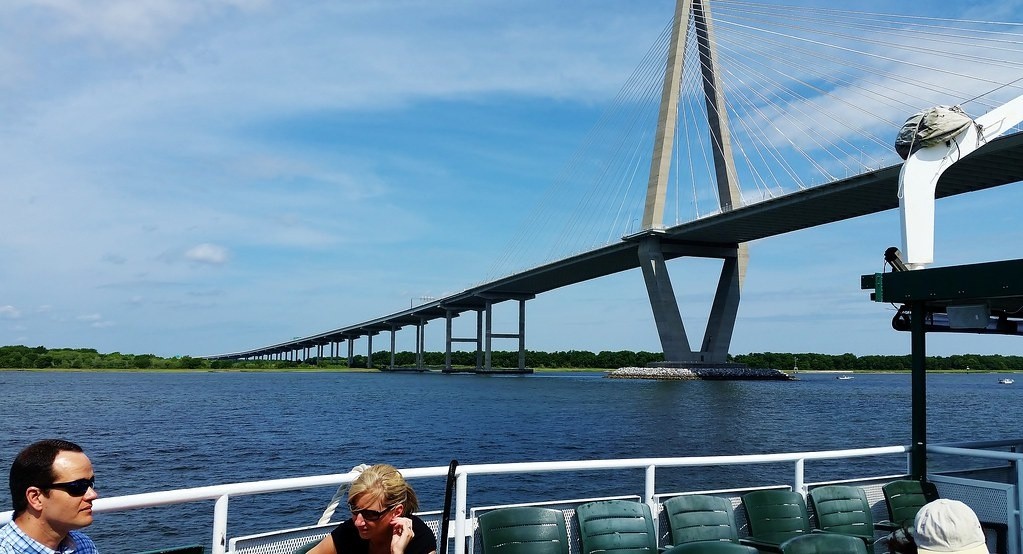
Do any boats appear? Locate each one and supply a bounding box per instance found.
[836,375,854,380]
[997,376,1014,384]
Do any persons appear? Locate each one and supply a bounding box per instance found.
[0,439,100,554]
[305,463,437,554]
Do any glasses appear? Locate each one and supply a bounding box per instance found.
[350,505,395,521]
[47,475,95,497]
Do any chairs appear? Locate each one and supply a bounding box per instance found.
[809,484,902,554]
[574,500,670,554]
[663,495,740,546]
[740,490,867,554]
[873,480,1008,554]
[477,506,569,554]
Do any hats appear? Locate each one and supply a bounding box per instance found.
[914,499,989,554]
